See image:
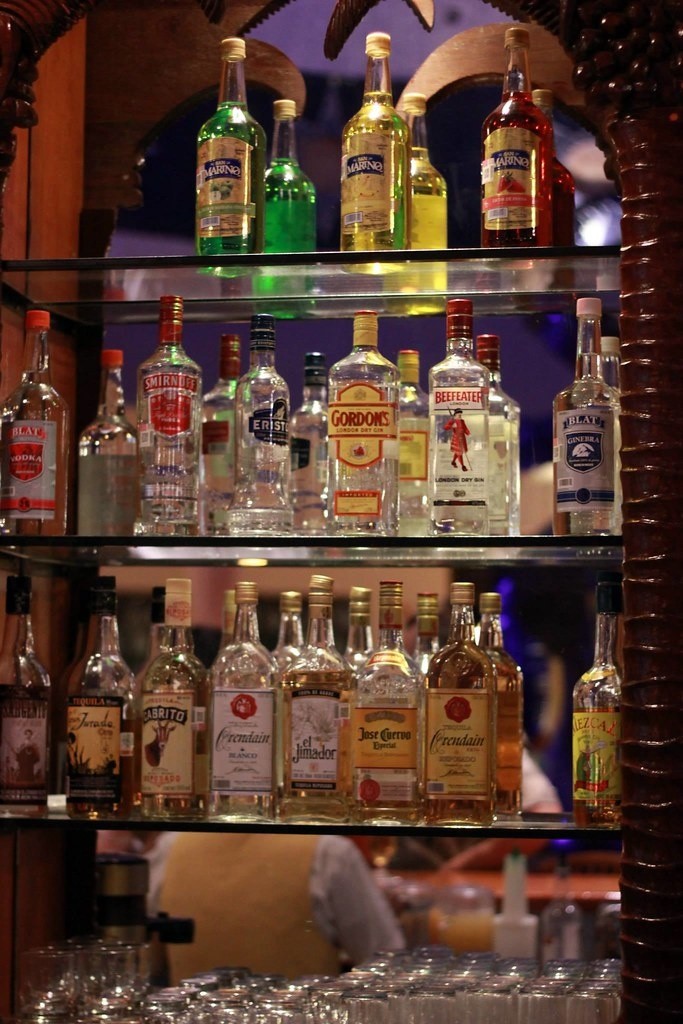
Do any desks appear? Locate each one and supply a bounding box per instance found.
[391,873,620,973]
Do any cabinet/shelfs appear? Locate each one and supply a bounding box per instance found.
[0,242,618,845]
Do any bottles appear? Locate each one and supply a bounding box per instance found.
[51,842,625,962]
[480,26,552,246]
[514,89,576,312]
[552,296,623,535]
[474,333,520,536]
[571,570,624,829]
[229,312,294,537]
[79,349,140,537]
[429,297,490,536]
[396,349,429,537]
[201,333,244,536]
[0,572,521,826]
[197,36,267,280]
[387,92,447,313]
[327,310,399,538]
[137,295,203,536]
[0,312,68,536]
[340,30,411,275]
[252,99,315,320]
[287,351,328,533]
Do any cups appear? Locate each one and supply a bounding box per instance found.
[12,939,624,1024]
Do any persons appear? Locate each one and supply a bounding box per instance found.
[348,750,564,870]
[14,728,41,780]
[142,832,407,984]
